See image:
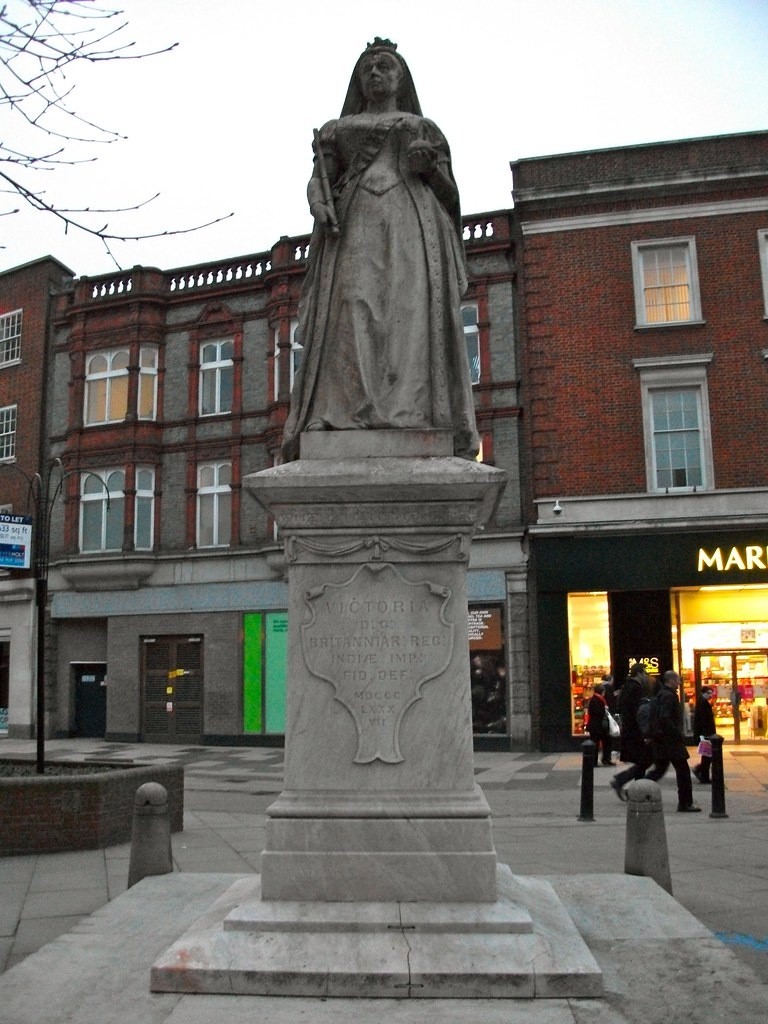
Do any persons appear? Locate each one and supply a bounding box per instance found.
[584,663,716,812]
[277,37,481,461]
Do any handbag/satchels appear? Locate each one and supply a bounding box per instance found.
[605,709,620,737]
[602,715,609,729]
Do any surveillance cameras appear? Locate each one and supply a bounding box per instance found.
[553,501,561,515]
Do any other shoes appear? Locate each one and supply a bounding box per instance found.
[692,767,700,779]
[678,804,700,812]
[602,759,615,765]
[610,779,625,801]
